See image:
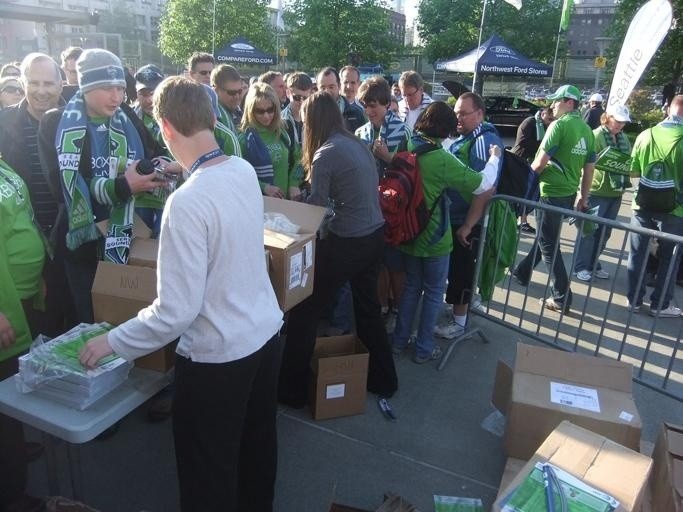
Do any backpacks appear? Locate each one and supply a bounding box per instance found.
[469,132,540,217]
[634,126,682,212]
[377,139,444,245]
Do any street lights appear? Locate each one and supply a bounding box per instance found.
[275,32,289,70]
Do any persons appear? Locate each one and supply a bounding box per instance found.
[0,253,45,510]
[0,46,682,411]
[77,74,288,510]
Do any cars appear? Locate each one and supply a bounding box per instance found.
[439,79,545,136]
[525,86,665,107]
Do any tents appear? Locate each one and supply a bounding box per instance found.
[433,33,553,96]
[214,37,277,67]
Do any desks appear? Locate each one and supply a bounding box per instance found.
[1,363,179,502]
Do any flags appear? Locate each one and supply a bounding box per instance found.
[606,0,673,115]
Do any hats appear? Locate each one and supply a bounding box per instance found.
[588,93,603,104]
[77,47,126,96]
[133,63,166,92]
[545,85,580,102]
[606,103,632,125]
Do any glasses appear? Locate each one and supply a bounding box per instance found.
[196,70,213,76]
[400,88,418,99]
[290,90,311,101]
[1,86,25,96]
[255,105,275,115]
[457,109,476,119]
[216,88,242,96]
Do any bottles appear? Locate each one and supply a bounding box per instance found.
[135,158,160,176]
[152,159,177,178]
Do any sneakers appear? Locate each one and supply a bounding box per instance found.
[649,304,682,317]
[595,269,610,278]
[626,299,641,311]
[517,222,538,234]
[384,294,484,364]
[506,266,529,285]
[573,269,591,281]
[539,296,571,314]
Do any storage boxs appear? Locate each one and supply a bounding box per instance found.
[89,193,330,372]
[307,334,370,421]
[489,341,683,512]
